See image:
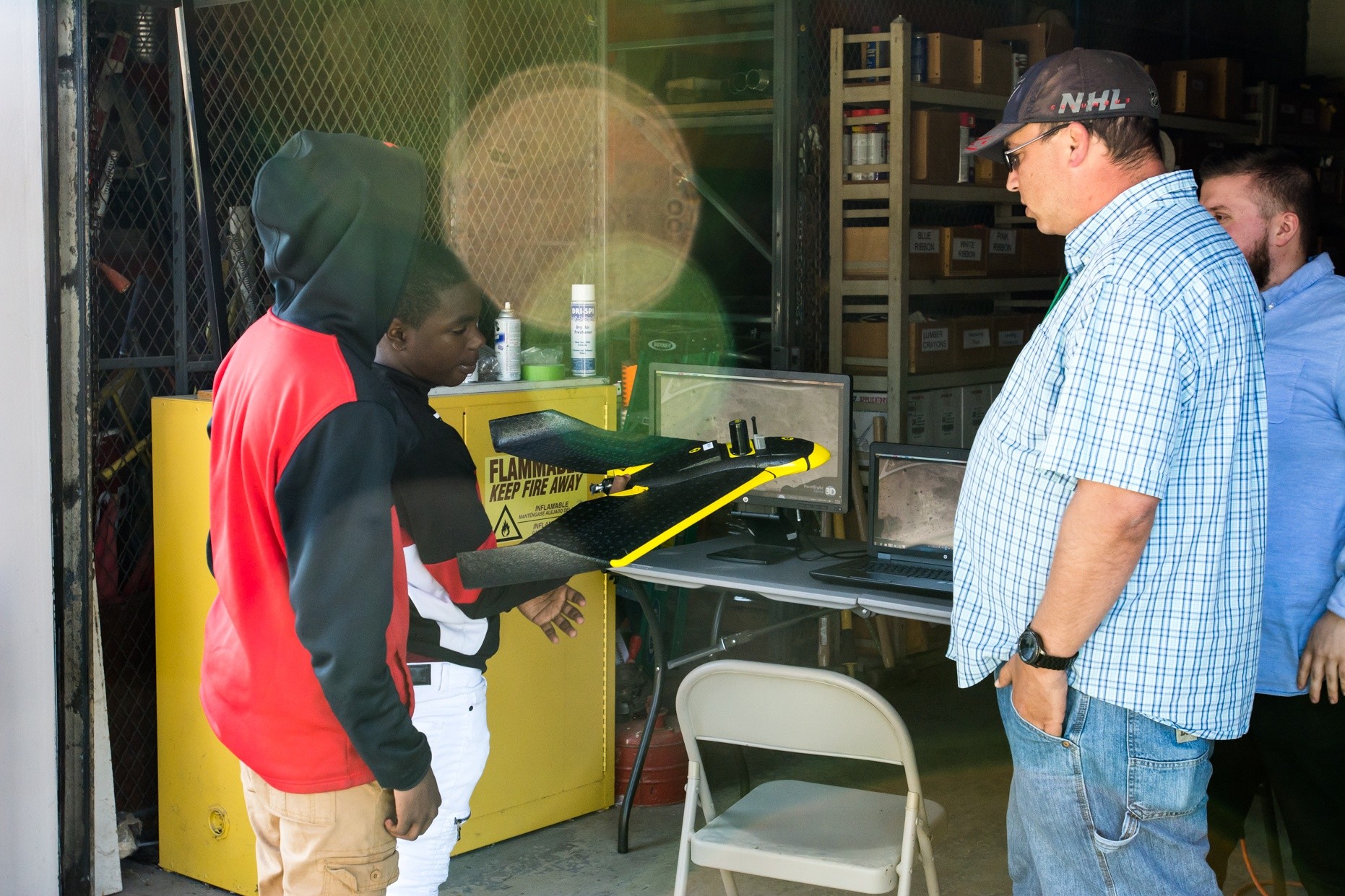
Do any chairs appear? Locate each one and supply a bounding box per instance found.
[675,660,946,896]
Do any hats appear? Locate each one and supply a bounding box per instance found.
[964,49,1161,155]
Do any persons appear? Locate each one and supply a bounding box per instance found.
[371,246,585,896]
[944,49,1271,896]
[199,129,442,896]
[1198,150,1345,896]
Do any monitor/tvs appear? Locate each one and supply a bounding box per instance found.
[648,362,854,564]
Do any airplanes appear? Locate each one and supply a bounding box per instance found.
[454,408,835,590]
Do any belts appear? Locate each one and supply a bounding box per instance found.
[406,663,431,686]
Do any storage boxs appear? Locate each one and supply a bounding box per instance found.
[842,26,1273,506]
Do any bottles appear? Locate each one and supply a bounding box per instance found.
[493,301,521,381]
[839,18,1035,183]
[478,349,499,382]
[570,284,598,377]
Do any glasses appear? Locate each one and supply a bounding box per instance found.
[1002,122,1092,173]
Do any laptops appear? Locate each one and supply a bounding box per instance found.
[807,441,973,599]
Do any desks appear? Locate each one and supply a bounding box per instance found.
[608,531,952,855]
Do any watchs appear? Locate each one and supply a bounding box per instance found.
[1018,621,1079,671]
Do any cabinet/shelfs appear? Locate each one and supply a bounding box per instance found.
[830,24,1293,650]
